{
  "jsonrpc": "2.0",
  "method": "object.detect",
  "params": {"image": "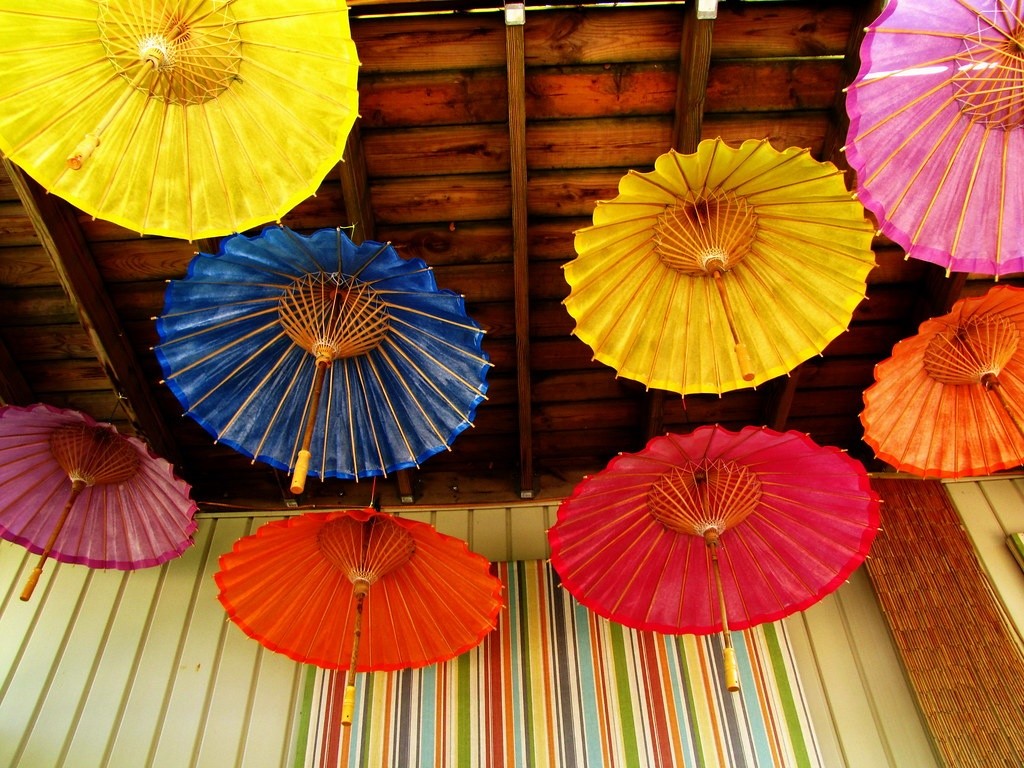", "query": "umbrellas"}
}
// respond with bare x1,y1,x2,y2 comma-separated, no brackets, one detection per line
0,403,201,602
838,0,1024,284
558,133,878,401
857,284,1024,479
543,422,886,694
150,224,495,496
214,507,509,727
0,0,363,243
